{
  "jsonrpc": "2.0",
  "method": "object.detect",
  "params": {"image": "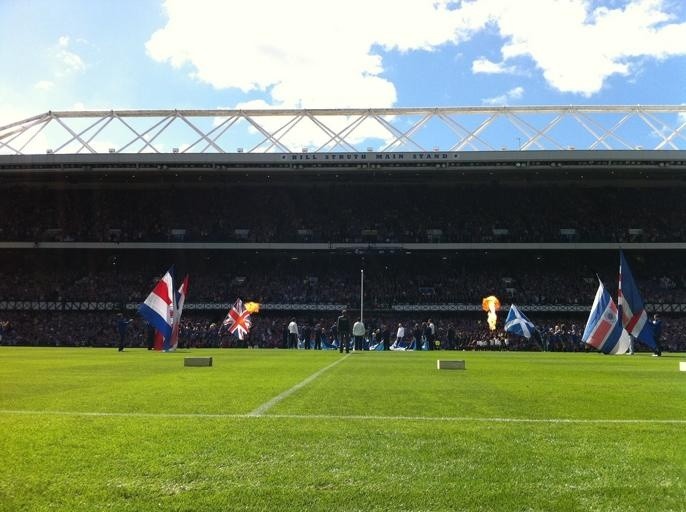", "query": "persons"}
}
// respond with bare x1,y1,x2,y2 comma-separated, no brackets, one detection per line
1,184,686,355
